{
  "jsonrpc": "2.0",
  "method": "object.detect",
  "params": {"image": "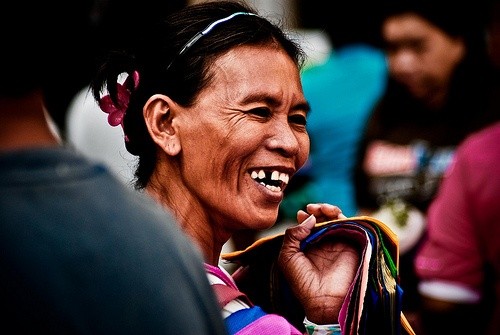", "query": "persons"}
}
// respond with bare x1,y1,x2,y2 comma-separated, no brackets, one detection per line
94,2,364,335
416,122,500,335
3,1,222,334
280,0,389,223
352,1,499,335
